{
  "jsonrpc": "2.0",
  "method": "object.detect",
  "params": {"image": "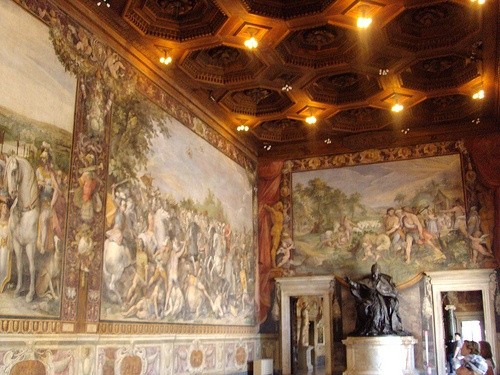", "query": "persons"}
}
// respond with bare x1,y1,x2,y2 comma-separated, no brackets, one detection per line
446,332,495,375
346,261,406,336
